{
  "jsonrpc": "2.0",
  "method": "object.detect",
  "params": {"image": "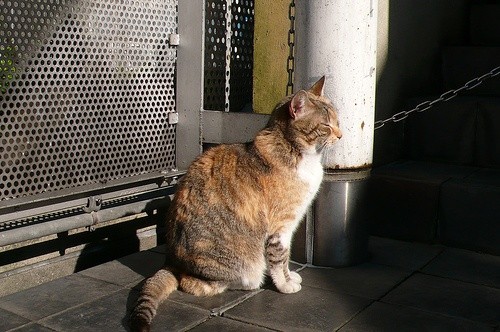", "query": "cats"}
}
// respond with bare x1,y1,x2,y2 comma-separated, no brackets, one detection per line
130,74,342,332
